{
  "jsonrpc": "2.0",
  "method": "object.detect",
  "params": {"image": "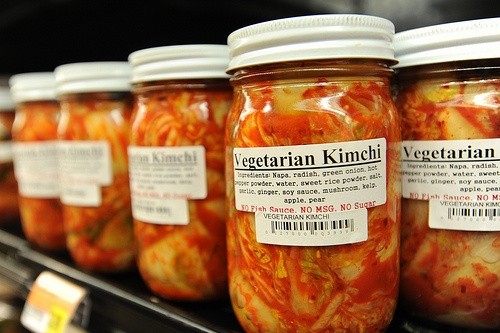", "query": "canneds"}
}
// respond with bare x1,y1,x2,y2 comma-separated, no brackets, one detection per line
1,13,500,332
